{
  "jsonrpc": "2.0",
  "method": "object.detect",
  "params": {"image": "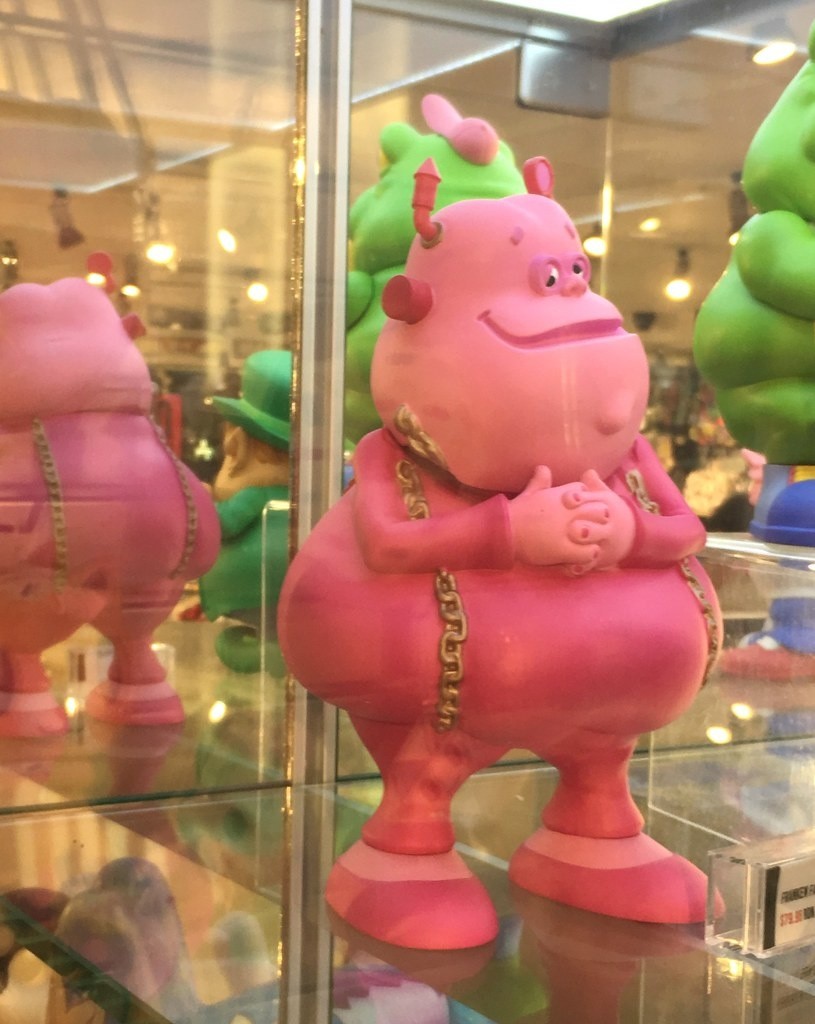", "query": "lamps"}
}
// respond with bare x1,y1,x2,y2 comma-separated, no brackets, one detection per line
0,12,797,300
